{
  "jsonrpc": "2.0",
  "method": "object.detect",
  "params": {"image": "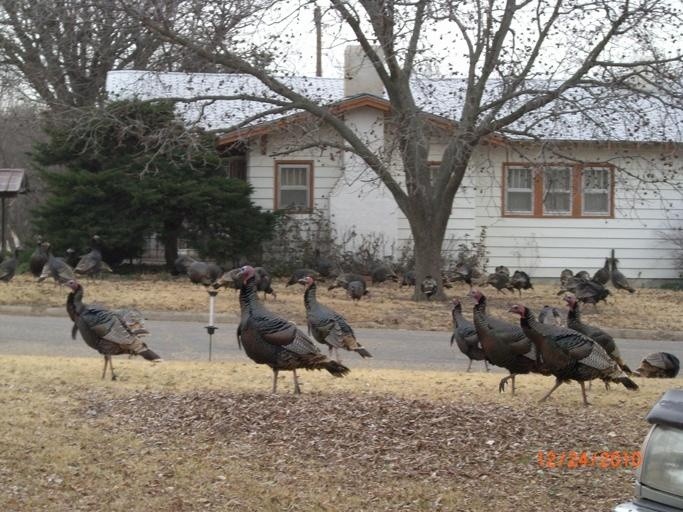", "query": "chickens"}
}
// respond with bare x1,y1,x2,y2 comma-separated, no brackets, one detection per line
421,273,438,303
62,279,161,382
173,250,415,396
0,244,26,284
449,257,640,407
634,351,680,379
29,233,113,288
